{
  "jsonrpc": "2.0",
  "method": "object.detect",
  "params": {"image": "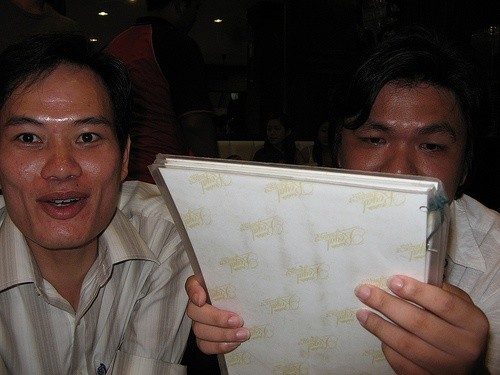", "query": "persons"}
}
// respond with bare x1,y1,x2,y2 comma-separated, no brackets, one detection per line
186,33,500,375
0,32,196,375
0,0,335,185
